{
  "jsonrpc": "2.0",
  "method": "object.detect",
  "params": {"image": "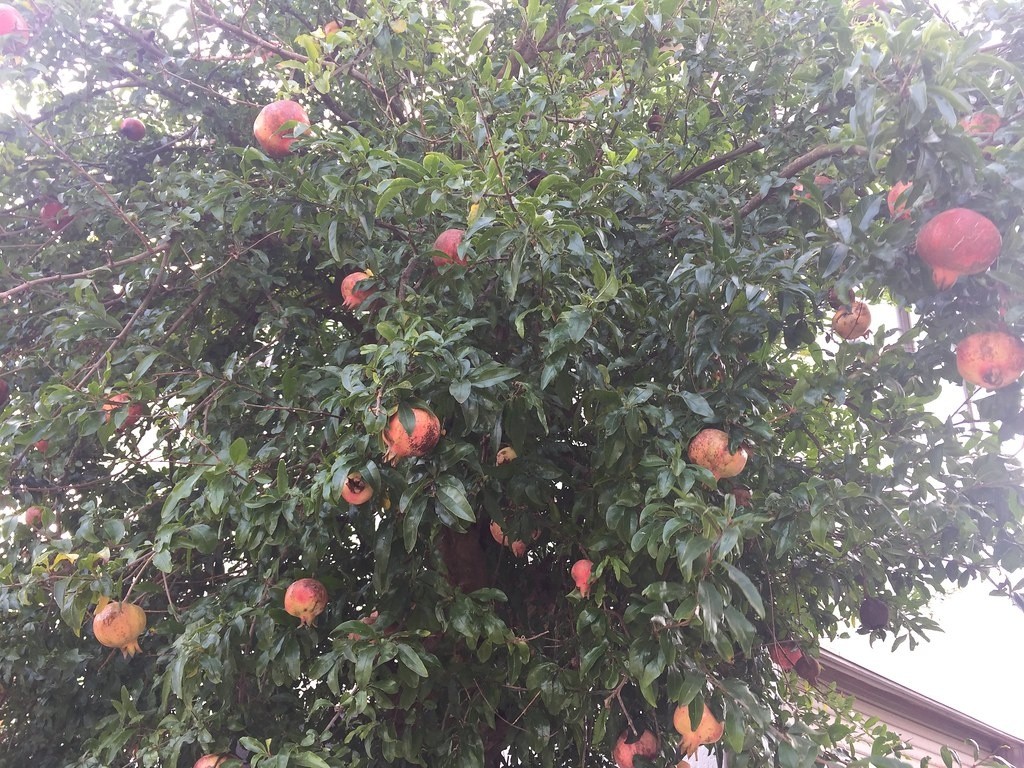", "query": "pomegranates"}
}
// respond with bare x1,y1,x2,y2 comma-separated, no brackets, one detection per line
0,0,1024,768
